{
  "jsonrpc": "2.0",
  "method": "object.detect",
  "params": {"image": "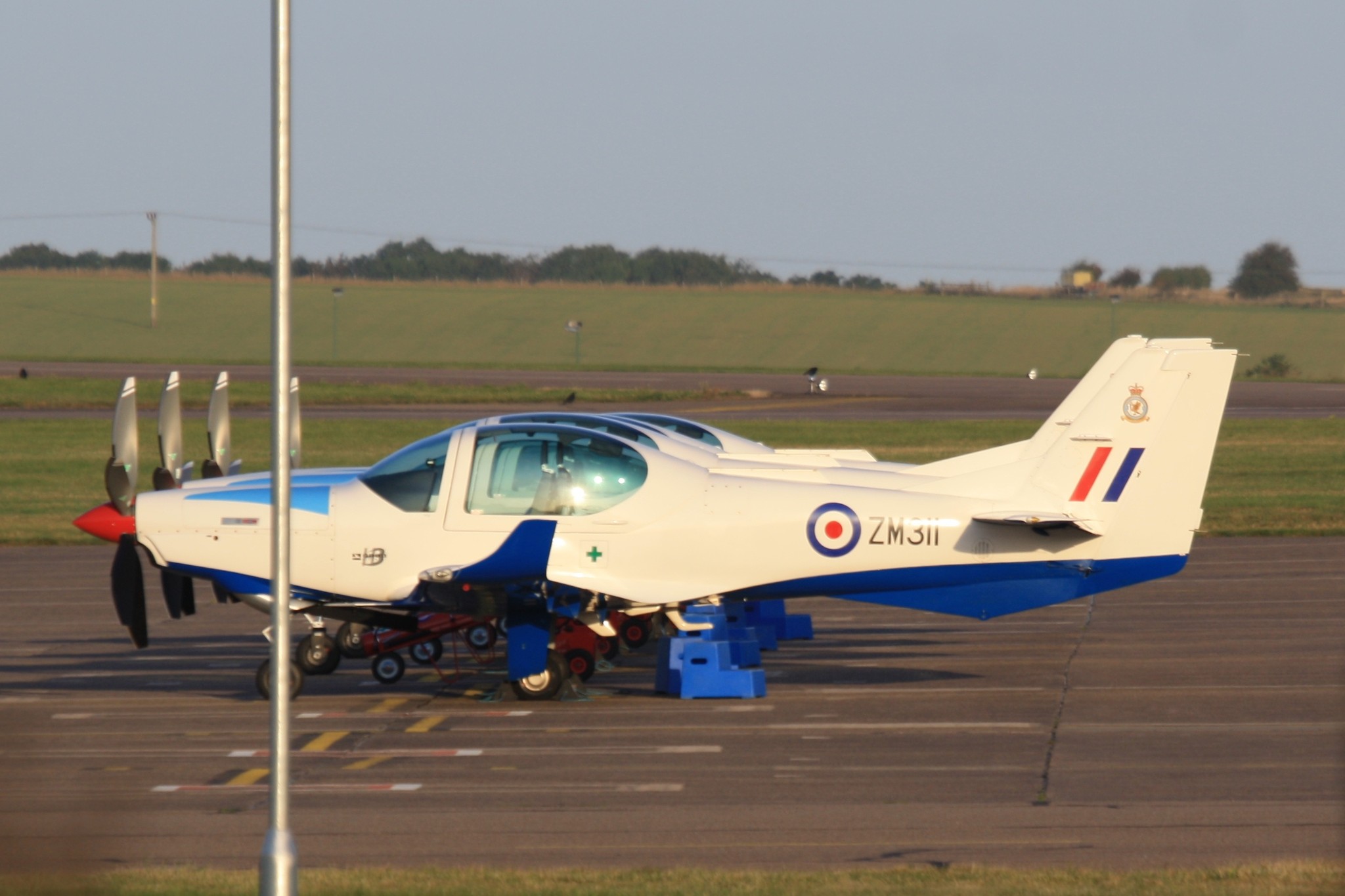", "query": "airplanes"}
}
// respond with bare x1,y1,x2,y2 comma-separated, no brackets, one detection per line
154,335,1217,682
74,347,1241,707
